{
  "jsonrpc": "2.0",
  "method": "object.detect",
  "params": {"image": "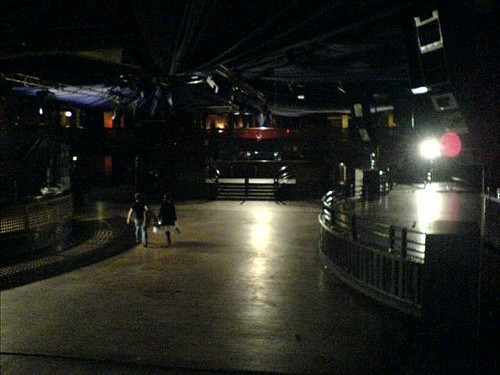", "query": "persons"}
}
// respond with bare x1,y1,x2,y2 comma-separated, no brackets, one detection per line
126,192,151,247
158,192,177,248
